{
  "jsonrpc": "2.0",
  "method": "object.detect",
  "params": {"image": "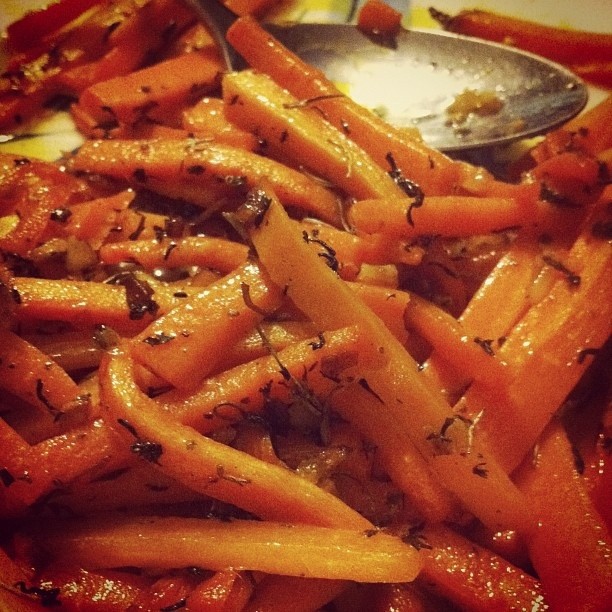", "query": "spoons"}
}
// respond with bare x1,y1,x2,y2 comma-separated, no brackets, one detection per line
191,1,590,153
